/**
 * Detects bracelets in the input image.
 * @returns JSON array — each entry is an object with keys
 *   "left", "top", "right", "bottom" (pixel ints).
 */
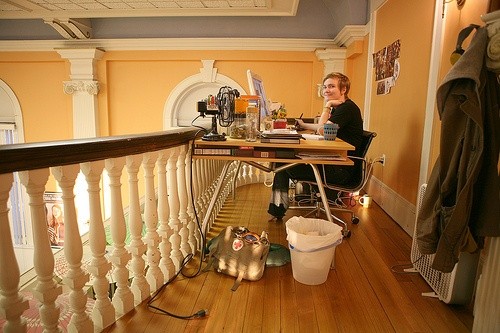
[{"left": 324, "top": 107, "right": 331, "bottom": 114}]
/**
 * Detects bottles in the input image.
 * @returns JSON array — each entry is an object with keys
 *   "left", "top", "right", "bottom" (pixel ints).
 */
[
  {"left": 246, "top": 103, "right": 259, "bottom": 142},
  {"left": 229, "top": 118, "right": 246, "bottom": 139}
]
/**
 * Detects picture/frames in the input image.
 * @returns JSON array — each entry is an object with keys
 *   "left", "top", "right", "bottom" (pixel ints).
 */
[{"left": 43, "top": 193, "right": 64, "bottom": 249}]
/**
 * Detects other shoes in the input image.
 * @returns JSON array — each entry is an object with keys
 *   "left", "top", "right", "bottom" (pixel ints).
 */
[{"left": 267, "top": 202, "right": 286, "bottom": 220}]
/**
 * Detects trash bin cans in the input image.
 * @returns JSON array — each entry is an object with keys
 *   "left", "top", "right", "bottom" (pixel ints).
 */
[{"left": 285, "top": 215, "right": 344, "bottom": 286}]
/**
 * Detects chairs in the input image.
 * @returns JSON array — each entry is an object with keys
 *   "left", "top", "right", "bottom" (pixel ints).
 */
[{"left": 268, "top": 130, "right": 377, "bottom": 239}]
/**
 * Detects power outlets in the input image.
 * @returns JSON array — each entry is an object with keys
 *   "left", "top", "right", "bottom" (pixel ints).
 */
[{"left": 380, "top": 154, "right": 386, "bottom": 166}]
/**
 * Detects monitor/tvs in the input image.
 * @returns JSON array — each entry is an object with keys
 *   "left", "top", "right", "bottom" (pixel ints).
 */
[{"left": 247, "top": 70, "right": 270, "bottom": 122}]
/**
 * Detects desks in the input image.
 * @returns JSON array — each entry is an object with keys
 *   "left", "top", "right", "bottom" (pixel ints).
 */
[{"left": 190, "top": 129, "right": 355, "bottom": 262}]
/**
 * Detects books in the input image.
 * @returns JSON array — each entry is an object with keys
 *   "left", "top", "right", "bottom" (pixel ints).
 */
[{"left": 261, "top": 133, "right": 302, "bottom": 143}]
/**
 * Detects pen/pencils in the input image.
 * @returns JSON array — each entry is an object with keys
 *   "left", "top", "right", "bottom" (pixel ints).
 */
[{"left": 296, "top": 113, "right": 303, "bottom": 125}]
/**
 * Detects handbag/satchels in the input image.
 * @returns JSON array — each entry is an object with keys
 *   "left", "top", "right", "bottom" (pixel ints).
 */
[{"left": 205, "top": 225, "right": 271, "bottom": 281}]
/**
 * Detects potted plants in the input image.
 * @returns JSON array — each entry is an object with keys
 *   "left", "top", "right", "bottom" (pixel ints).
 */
[{"left": 272, "top": 106, "right": 287, "bottom": 129}]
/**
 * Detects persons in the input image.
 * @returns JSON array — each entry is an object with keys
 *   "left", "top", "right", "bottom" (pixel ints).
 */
[
  {"left": 50, "top": 205, "right": 65, "bottom": 241},
  {"left": 44, "top": 207, "right": 60, "bottom": 244},
  {"left": 267, "top": 72, "right": 364, "bottom": 222}
]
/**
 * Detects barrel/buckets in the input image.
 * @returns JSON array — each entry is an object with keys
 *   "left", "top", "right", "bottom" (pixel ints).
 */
[{"left": 285, "top": 218, "right": 342, "bottom": 285}]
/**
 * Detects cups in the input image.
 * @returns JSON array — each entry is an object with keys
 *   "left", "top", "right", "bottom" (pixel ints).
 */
[
  {"left": 274, "top": 120, "right": 288, "bottom": 130},
  {"left": 318, "top": 124, "right": 337, "bottom": 141},
  {"left": 359, "top": 194, "right": 372, "bottom": 209}
]
